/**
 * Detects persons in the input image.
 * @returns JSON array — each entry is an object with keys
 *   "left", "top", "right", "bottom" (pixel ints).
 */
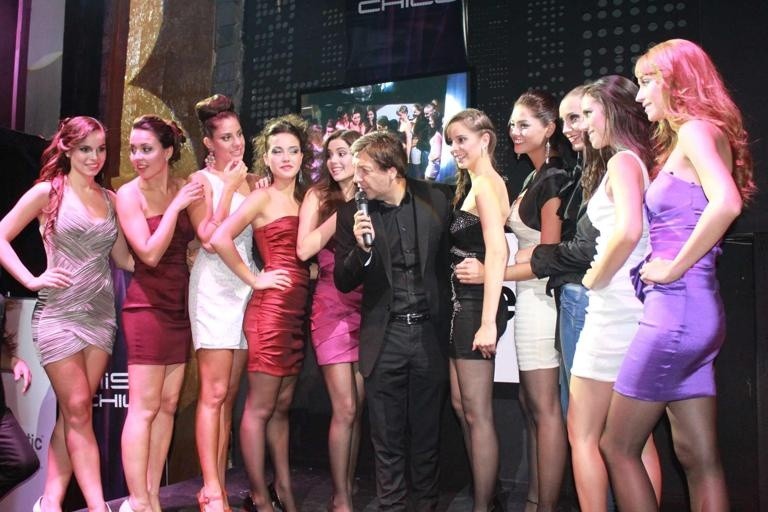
[{"left": 1, "top": 38, "right": 757, "bottom": 512}]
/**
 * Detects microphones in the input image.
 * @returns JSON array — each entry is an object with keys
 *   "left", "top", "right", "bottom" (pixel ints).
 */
[{"left": 353, "top": 190, "right": 375, "bottom": 249}]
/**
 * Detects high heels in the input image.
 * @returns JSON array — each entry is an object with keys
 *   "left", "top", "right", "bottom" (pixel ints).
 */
[
  {"left": 196, "top": 485, "right": 233, "bottom": 512},
  {"left": 267, "top": 483, "right": 286, "bottom": 512},
  {"left": 244, "top": 493, "right": 260, "bottom": 512}
]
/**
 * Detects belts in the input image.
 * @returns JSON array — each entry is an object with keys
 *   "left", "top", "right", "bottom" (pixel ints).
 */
[{"left": 391, "top": 311, "right": 432, "bottom": 325}]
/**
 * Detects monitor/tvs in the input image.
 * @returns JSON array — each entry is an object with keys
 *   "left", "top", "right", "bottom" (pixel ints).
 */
[{"left": 294, "top": 66, "right": 474, "bottom": 194}]
